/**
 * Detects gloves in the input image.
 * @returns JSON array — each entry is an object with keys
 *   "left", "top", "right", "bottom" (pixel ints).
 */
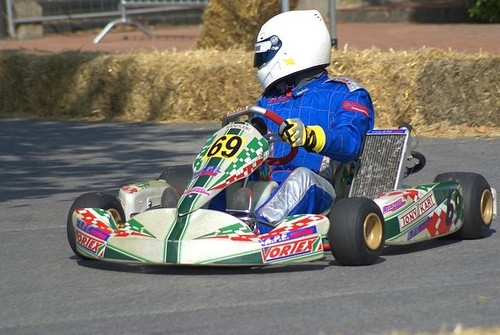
[
  {"left": 279, "top": 118, "right": 326, "bottom": 154},
  {"left": 236, "top": 112, "right": 249, "bottom": 122}
]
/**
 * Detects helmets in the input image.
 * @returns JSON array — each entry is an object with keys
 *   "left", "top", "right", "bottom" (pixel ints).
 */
[{"left": 253, "top": 10, "right": 332, "bottom": 92}]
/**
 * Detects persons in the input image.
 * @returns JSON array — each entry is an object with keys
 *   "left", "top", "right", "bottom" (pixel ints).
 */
[{"left": 200, "top": 9, "right": 375, "bottom": 235}]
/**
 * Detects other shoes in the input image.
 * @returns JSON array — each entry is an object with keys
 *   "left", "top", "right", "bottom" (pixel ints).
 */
[
  {"left": 161, "top": 187, "right": 178, "bottom": 208},
  {"left": 229, "top": 187, "right": 255, "bottom": 232}
]
